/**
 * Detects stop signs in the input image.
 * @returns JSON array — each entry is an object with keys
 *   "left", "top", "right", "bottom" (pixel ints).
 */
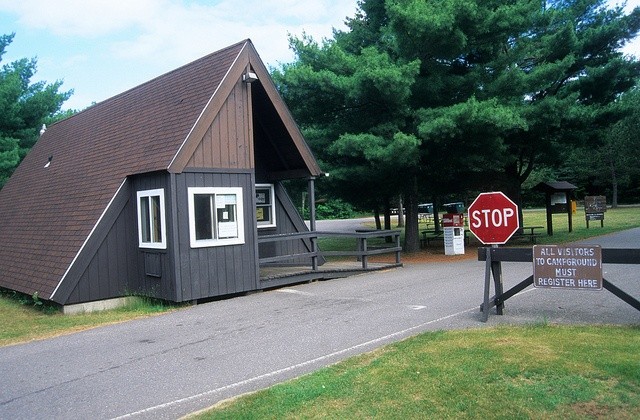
[{"left": 468, "top": 191, "right": 519, "bottom": 244}]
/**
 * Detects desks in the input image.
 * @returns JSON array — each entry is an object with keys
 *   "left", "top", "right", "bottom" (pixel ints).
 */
[
  {"left": 420, "top": 230, "right": 470, "bottom": 237},
  {"left": 518, "top": 226, "right": 544, "bottom": 234}
]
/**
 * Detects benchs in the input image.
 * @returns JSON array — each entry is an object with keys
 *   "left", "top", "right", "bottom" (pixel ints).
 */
[
  {"left": 420, "top": 238, "right": 465, "bottom": 249},
  {"left": 426, "top": 222, "right": 444, "bottom": 230},
  {"left": 509, "top": 234, "right": 543, "bottom": 245}
]
